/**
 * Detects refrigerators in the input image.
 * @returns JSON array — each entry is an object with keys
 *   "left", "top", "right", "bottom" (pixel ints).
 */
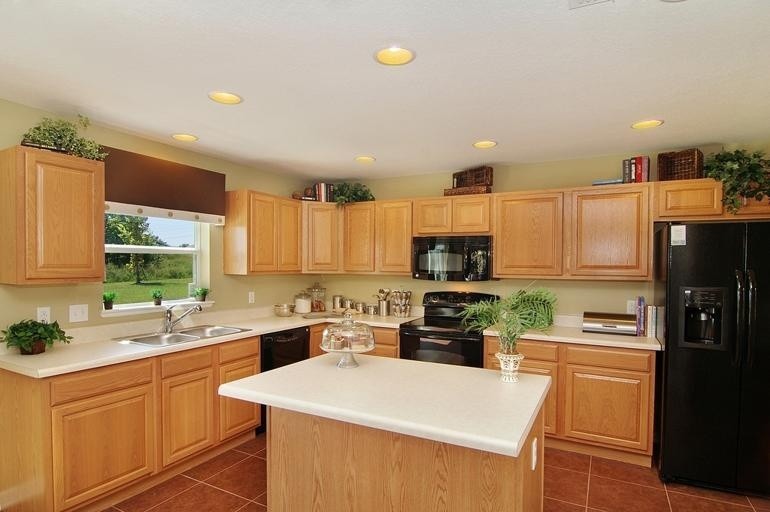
[{"left": 651, "top": 221, "right": 770, "bottom": 498}]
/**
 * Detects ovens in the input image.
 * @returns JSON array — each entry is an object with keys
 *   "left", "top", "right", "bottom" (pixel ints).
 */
[{"left": 398, "top": 332, "right": 483, "bottom": 367}]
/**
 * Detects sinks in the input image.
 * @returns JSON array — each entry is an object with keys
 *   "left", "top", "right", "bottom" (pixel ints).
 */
[
  {"left": 177, "top": 325, "right": 253, "bottom": 344},
  {"left": 111, "top": 331, "right": 200, "bottom": 354}
]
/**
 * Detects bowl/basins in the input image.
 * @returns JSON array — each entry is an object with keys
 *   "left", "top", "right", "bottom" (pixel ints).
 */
[{"left": 274, "top": 304, "right": 296, "bottom": 316}]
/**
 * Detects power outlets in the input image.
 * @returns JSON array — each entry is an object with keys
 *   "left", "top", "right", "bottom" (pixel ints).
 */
[{"left": 36, "top": 307, "right": 50, "bottom": 324}]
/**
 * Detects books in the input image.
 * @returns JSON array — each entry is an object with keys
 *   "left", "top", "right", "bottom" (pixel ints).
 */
[
  {"left": 299, "top": 182, "right": 334, "bottom": 203},
  {"left": 636, "top": 295, "right": 656, "bottom": 338},
  {"left": 622, "top": 155, "right": 650, "bottom": 183},
  {"left": 21, "top": 142, "right": 66, "bottom": 152},
  {"left": 592, "top": 179, "right": 622, "bottom": 186}
]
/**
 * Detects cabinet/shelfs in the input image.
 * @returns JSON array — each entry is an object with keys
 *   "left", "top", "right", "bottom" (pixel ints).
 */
[
  {"left": 0, "top": 358, "right": 158, "bottom": 512},
  {"left": 223, "top": 189, "right": 305, "bottom": 276},
  {"left": 483, "top": 336, "right": 656, "bottom": 457},
  {"left": 310, "top": 324, "right": 398, "bottom": 359},
  {"left": 0, "top": 145, "right": 105, "bottom": 286},
  {"left": 305, "top": 200, "right": 344, "bottom": 275},
  {"left": 158, "top": 337, "right": 261, "bottom": 468}
]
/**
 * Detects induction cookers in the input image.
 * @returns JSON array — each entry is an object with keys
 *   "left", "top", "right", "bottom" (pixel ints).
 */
[{"left": 400, "top": 316, "right": 483, "bottom": 336}]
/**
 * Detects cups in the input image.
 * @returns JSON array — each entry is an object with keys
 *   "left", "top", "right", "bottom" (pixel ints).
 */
[{"left": 377, "top": 300, "right": 390, "bottom": 315}]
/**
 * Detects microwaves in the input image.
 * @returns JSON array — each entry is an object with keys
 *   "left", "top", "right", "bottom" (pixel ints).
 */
[{"left": 411, "top": 235, "right": 500, "bottom": 282}]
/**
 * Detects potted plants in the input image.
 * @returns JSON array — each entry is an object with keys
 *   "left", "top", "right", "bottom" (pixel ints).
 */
[
  {"left": 0, "top": 320, "right": 73, "bottom": 355},
  {"left": 454, "top": 288, "right": 558, "bottom": 384},
  {"left": 103, "top": 292, "right": 116, "bottom": 310},
  {"left": 151, "top": 289, "right": 163, "bottom": 305},
  {"left": 191, "top": 288, "right": 208, "bottom": 301}
]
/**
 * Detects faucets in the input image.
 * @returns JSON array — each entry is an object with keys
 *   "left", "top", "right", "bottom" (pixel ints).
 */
[{"left": 165, "top": 304, "right": 203, "bottom": 333}]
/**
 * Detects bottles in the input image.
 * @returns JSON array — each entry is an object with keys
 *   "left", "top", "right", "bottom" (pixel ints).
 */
[
  {"left": 307, "top": 281, "right": 327, "bottom": 313},
  {"left": 392, "top": 290, "right": 411, "bottom": 317},
  {"left": 294, "top": 290, "right": 312, "bottom": 314},
  {"left": 332, "top": 294, "right": 378, "bottom": 316}
]
